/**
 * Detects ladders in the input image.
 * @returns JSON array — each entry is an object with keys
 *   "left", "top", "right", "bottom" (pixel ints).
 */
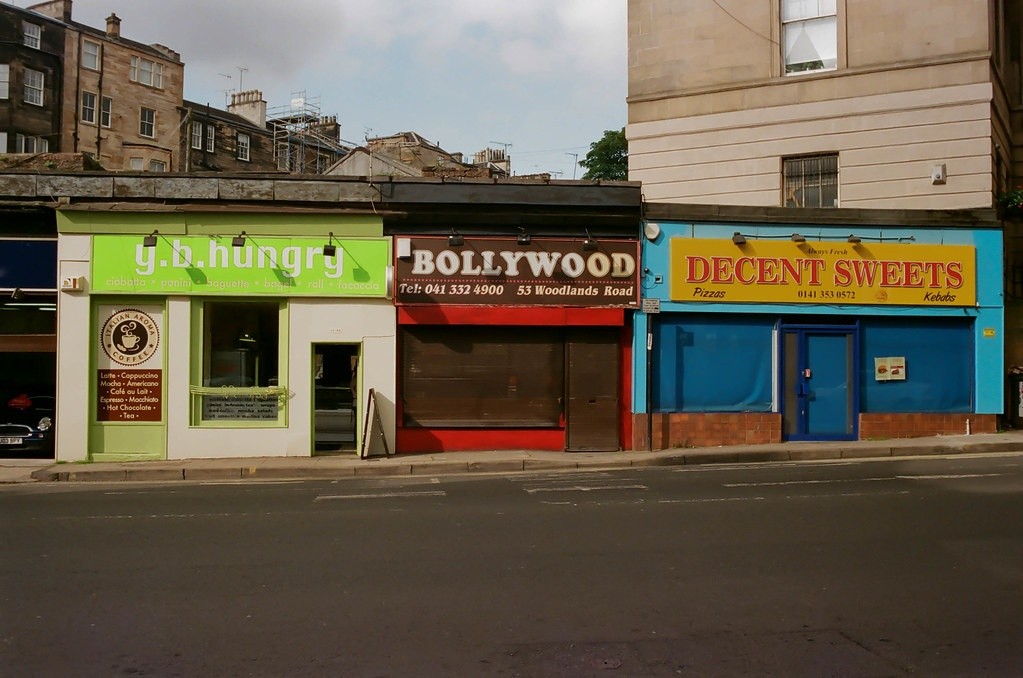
[{"left": 360, "top": 388, "right": 392, "bottom": 460}]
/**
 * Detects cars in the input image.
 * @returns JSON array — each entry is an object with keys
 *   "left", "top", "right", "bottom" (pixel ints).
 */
[{"left": 0, "top": 396, "right": 56, "bottom": 451}]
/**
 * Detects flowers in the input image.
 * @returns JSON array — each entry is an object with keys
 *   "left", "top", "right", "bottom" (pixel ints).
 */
[{"left": 998, "top": 184, "right": 1023, "bottom": 209}]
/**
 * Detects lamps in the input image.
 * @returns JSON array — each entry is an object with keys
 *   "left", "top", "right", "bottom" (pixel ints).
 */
[{"left": 143, "top": 222, "right": 862, "bottom": 256}]
